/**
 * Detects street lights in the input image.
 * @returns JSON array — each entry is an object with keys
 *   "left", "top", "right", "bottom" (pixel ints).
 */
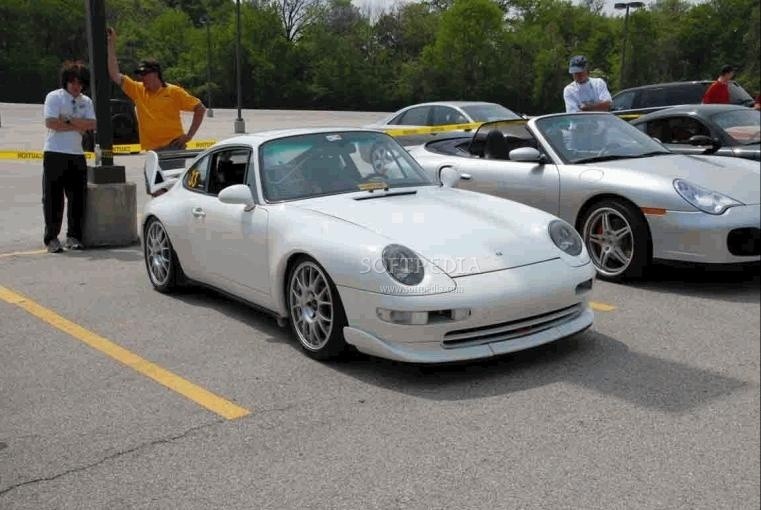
[{"left": 615, "top": 2, "right": 645, "bottom": 81}]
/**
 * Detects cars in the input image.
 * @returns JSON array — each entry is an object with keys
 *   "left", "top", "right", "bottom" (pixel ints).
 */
[{"left": 140, "top": 80, "right": 761, "bottom": 363}]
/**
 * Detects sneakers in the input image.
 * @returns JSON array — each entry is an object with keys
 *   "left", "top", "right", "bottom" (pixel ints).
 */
[
  {"left": 66, "top": 237, "right": 85, "bottom": 251},
  {"left": 47, "top": 236, "right": 65, "bottom": 253}
]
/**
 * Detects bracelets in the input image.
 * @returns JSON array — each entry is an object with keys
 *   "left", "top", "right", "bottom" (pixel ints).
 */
[{"left": 65, "top": 116, "right": 71, "bottom": 124}]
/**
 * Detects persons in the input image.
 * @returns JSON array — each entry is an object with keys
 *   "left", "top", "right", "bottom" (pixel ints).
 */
[
  {"left": 106, "top": 26, "right": 208, "bottom": 196},
  {"left": 702, "top": 64, "right": 740, "bottom": 104},
  {"left": 41, "top": 64, "right": 98, "bottom": 254},
  {"left": 563, "top": 55, "right": 614, "bottom": 150},
  {"left": 755, "top": 90, "right": 761, "bottom": 111}
]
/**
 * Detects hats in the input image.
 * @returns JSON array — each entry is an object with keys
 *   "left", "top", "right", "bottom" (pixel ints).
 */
[
  {"left": 132, "top": 58, "right": 162, "bottom": 75},
  {"left": 567, "top": 55, "right": 591, "bottom": 74}
]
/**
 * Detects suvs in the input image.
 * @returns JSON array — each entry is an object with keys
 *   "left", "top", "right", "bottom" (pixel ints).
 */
[{"left": 82, "top": 98, "right": 139, "bottom": 151}]
